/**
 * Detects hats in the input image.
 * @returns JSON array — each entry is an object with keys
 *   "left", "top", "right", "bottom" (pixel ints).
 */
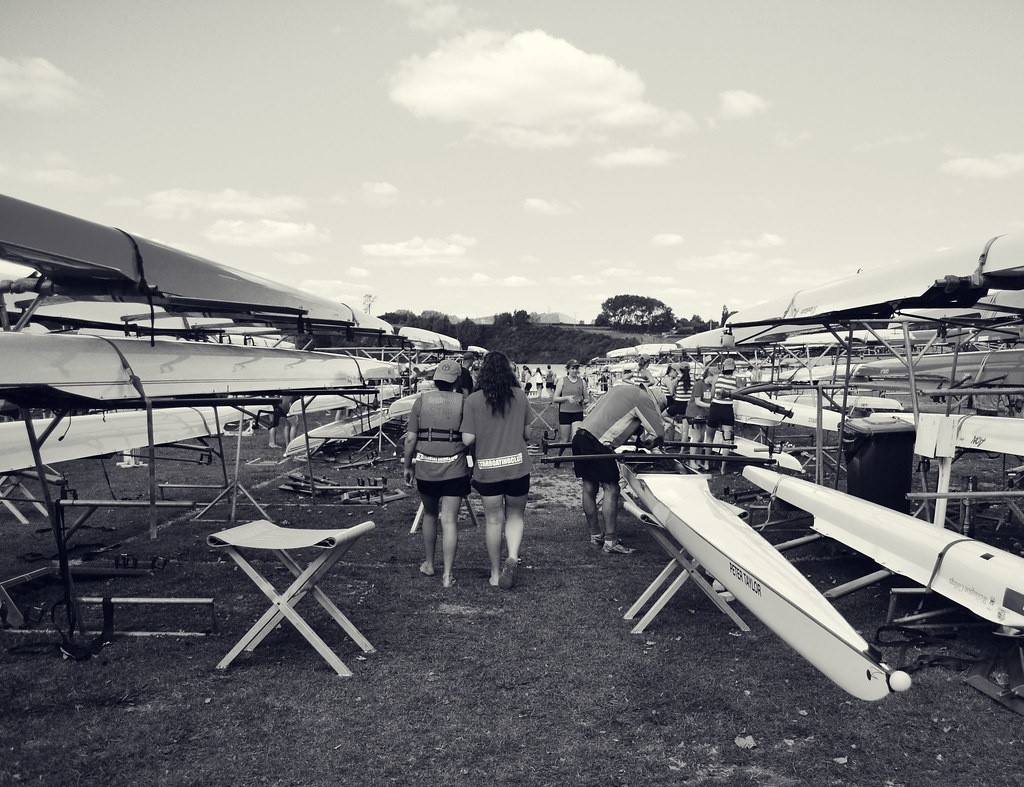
[
  {"left": 639, "top": 354, "right": 735, "bottom": 376},
  {"left": 566, "top": 359, "right": 580, "bottom": 366},
  {"left": 433, "top": 360, "right": 461, "bottom": 383},
  {"left": 641, "top": 384, "right": 667, "bottom": 418},
  {"left": 464, "top": 353, "right": 475, "bottom": 361}
]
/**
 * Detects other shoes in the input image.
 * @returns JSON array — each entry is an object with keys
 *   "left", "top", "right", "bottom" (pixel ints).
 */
[
  {"left": 420, "top": 562, "right": 435, "bottom": 576},
  {"left": 442, "top": 573, "right": 456, "bottom": 587}
]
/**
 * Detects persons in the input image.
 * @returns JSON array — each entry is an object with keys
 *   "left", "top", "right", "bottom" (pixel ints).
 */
[
  {"left": 571, "top": 381, "right": 669, "bottom": 556},
  {"left": 701, "top": 357, "right": 746, "bottom": 475},
  {"left": 402, "top": 359, "right": 474, "bottom": 588},
  {"left": 552, "top": 358, "right": 589, "bottom": 469},
  {"left": 500, "top": 350, "right": 764, "bottom": 410},
  {"left": 683, "top": 366, "right": 723, "bottom": 472},
  {"left": 264, "top": 385, "right": 305, "bottom": 454},
  {"left": 458, "top": 351, "right": 536, "bottom": 586},
  {"left": 453, "top": 350, "right": 477, "bottom": 404},
  {"left": 677, "top": 373, "right": 712, "bottom": 465}
]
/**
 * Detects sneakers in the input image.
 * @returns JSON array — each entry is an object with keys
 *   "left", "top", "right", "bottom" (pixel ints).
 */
[
  {"left": 498, "top": 555, "right": 518, "bottom": 589},
  {"left": 488, "top": 571, "right": 499, "bottom": 586},
  {"left": 602, "top": 539, "right": 637, "bottom": 556},
  {"left": 590, "top": 531, "right": 605, "bottom": 549}
]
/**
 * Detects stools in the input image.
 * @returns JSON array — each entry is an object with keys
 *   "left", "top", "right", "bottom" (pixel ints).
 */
[
  {"left": 200, "top": 518, "right": 377, "bottom": 677},
  {"left": 527, "top": 400, "right": 555, "bottom": 430}
]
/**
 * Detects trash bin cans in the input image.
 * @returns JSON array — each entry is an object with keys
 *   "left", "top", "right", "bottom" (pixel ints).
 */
[{"left": 838, "top": 412, "right": 917, "bottom": 516}]
